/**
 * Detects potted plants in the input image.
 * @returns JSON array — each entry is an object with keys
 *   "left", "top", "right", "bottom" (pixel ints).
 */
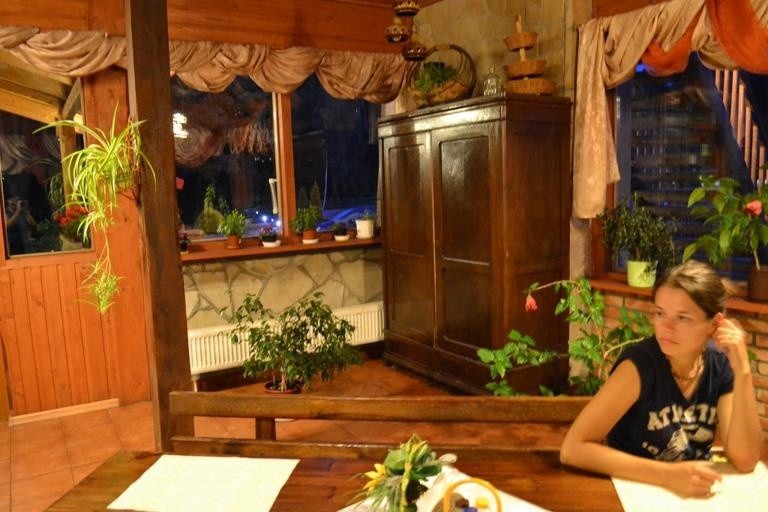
[
  {"left": 590, "top": 188, "right": 686, "bottom": 289},
  {"left": 212, "top": 288, "right": 366, "bottom": 422},
  {"left": 30, "top": 95, "right": 159, "bottom": 317},
  {"left": 214, "top": 199, "right": 377, "bottom": 252}
]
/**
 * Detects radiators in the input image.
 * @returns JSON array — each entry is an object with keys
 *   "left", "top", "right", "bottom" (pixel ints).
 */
[{"left": 186, "top": 302, "right": 385, "bottom": 392}]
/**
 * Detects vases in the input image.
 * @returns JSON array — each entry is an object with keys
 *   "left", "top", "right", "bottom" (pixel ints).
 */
[{"left": 747, "top": 264, "right": 768, "bottom": 304}]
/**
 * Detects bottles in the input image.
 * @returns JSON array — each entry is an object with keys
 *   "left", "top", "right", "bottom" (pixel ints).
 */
[{"left": 482, "top": 66, "right": 503, "bottom": 98}]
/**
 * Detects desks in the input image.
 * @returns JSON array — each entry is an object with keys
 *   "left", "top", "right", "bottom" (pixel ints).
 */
[{"left": 40, "top": 450, "right": 768, "bottom": 512}]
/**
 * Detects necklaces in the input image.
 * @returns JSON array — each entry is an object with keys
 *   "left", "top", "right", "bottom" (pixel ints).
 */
[{"left": 669, "top": 355, "right": 703, "bottom": 381}]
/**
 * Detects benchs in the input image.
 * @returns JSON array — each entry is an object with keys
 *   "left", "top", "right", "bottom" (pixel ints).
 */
[{"left": 168, "top": 390, "right": 606, "bottom": 459}]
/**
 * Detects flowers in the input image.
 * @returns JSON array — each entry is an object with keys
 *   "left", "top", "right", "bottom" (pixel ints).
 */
[
  {"left": 475, "top": 275, "right": 656, "bottom": 399},
  {"left": 683, "top": 173, "right": 768, "bottom": 269}
]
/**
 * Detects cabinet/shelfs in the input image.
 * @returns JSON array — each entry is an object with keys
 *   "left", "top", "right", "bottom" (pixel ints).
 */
[{"left": 377, "top": 91, "right": 577, "bottom": 396}]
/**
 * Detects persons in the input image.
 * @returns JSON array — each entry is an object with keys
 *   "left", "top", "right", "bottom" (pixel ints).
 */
[
  {"left": 558, "top": 258, "right": 764, "bottom": 499},
  {"left": 6, "top": 191, "right": 38, "bottom": 252}
]
